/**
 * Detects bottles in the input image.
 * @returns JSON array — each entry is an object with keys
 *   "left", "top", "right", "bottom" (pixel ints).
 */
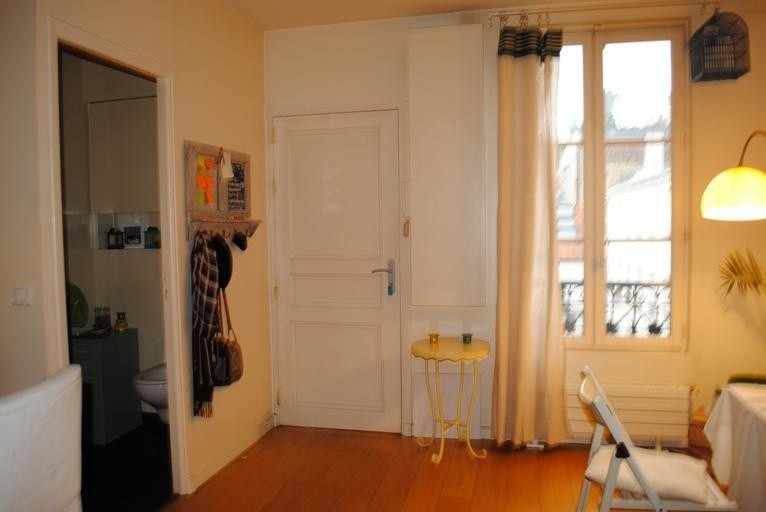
[{"left": 92, "top": 303, "right": 127, "bottom": 335}]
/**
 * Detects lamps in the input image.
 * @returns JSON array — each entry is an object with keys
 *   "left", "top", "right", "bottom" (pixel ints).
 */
[{"left": 700, "top": 123, "right": 766, "bottom": 221}]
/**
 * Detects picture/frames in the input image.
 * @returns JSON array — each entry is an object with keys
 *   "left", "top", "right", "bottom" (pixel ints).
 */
[{"left": 183, "top": 140, "right": 254, "bottom": 221}]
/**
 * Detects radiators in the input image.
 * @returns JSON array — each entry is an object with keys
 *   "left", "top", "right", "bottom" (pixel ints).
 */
[{"left": 563, "top": 378, "right": 691, "bottom": 448}]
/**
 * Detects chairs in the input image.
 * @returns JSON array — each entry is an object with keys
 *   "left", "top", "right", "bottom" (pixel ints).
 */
[{"left": 574, "top": 362, "right": 739, "bottom": 512}]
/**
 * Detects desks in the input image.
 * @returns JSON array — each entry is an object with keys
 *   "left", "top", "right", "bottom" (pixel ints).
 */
[
  {"left": 409, "top": 334, "right": 496, "bottom": 466},
  {"left": 700, "top": 378, "right": 765, "bottom": 512}
]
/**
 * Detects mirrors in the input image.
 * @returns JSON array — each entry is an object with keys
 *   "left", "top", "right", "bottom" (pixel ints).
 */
[{"left": 83, "top": 93, "right": 160, "bottom": 246}]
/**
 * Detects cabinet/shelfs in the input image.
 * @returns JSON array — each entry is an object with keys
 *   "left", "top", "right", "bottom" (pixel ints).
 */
[{"left": 66, "top": 324, "right": 143, "bottom": 451}]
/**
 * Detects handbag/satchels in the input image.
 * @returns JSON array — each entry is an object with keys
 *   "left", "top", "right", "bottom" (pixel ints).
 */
[{"left": 211, "top": 336, "right": 244, "bottom": 386}]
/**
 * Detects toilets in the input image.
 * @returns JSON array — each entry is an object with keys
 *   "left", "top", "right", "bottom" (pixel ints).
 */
[{"left": 131, "top": 363, "right": 170, "bottom": 449}]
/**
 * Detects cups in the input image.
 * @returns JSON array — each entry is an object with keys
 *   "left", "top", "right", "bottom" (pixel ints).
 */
[
  {"left": 428, "top": 333, "right": 439, "bottom": 345},
  {"left": 462, "top": 334, "right": 474, "bottom": 345}
]
